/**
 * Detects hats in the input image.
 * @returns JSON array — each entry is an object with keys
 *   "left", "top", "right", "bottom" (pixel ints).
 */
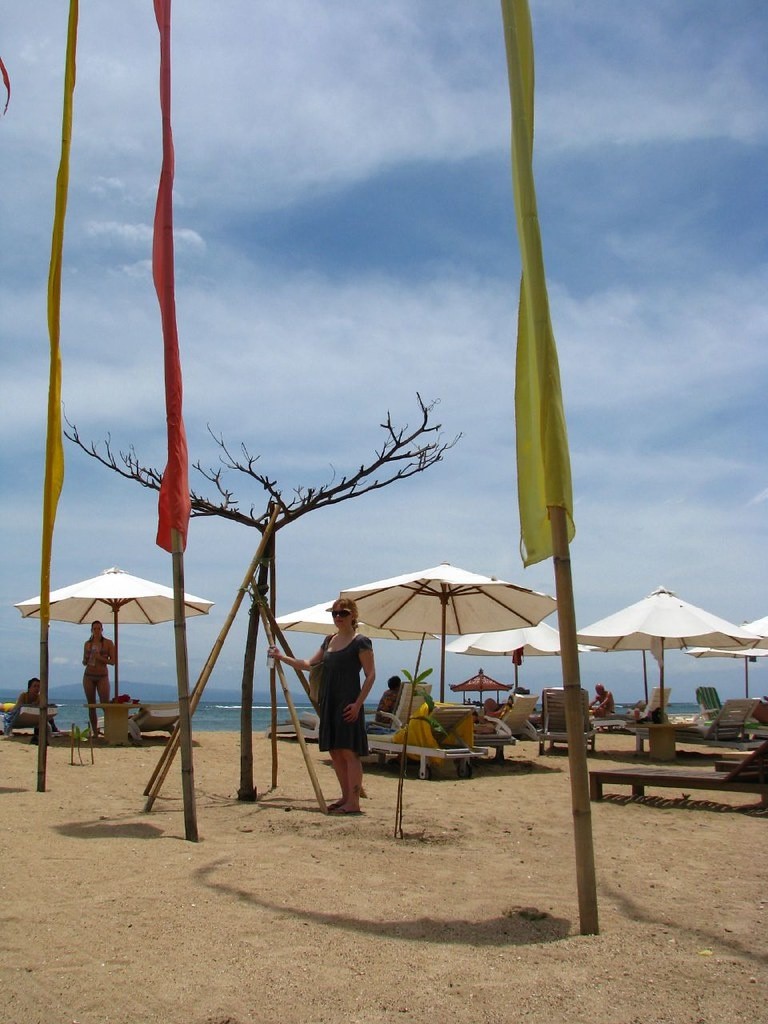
[
  {"left": 388, "top": 676, "right": 401, "bottom": 687},
  {"left": 514, "top": 687, "right": 530, "bottom": 695}
]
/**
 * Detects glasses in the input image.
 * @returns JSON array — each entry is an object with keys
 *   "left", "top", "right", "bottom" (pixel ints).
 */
[{"left": 332, "top": 610, "right": 353, "bottom": 617}]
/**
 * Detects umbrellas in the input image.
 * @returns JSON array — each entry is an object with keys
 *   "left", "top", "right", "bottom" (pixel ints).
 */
[
  {"left": 586, "top": 644, "right": 650, "bottom": 706},
  {"left": 444, "top": 623, "right": 592, "bottom": 658},
  {"left": 267, "top": 597, "right": 440, "bottom": 641},
  {"left": 326, "top": 561, "right": 558, "bottom": 703},
  {"left": 739, "top": 614, "right": 768, "bottom": 649},
  {"left": 14, "top": 567, "right": 215, "bottom": 698},
  {"left": 684, "top": 645, "right": 768, "bottom": 699},
  {"left": 575, "top": 585, "right": 765, "bottom": 723}
]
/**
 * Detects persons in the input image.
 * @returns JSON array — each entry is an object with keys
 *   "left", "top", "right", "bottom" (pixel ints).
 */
[
  {"left": 588, "top": 684, "right": 616, "bottom": 732},
  {"left": 267, "top": 597, "right": 375, "bottom": 816},
  {"left": 374, "top": 676, "right": 402, "bottom": 724},
  {"left": 632, "top": 707, "right": 661, "bottom": 723},
  {"left": 80, "top": 620, "right": 116, "bottom": 738},
  {"left": 467, "top": 697, "right": 511, "bottom": 723},
  {"left": 0, "top": 678, "right": 41, "bottom": 713}
]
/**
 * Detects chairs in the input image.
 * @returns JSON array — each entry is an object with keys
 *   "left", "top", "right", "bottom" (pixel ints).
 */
[
  {"left": 474, "top": 729, "right": 516, "bottom": 764},
  {"left": 367, "top": 701, "right": 496, "bottom": 780},
  {"left": 539, "top": 687, "right": 597, "bottom": 755},
  {"left": 366, "top": 682, "right": 432, "bottom": 730},
  {"left": 97, "top": 705, "right": 179, "bottom": 740},
  {"left": 266, "top": 712, "right": 319, "bottom": 739},
  {"left": 589, "top": 739, "right": 768, "bottom": 802},
  {"left": 635, "top": 698, "right": 768, "bottom": 752},
  {"left": 495, "top": 694, "right": 539, "bottom": 735},
  {"left": 0, "top": 703, "right": 58, "bottom": 746},
  {"left": 592, "top": 687, "right": 671, "bottom": 732},
  {"left": 696, "top": 685, "right": 768, "bottom": 736}
]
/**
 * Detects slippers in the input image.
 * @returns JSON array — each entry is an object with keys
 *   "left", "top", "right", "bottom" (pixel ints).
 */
[
  {"left": 332, "top": 809, "right": 361, "bottom": 815},
  {"left": 327, "top": 802, "right": 340, "bottom": 811}
]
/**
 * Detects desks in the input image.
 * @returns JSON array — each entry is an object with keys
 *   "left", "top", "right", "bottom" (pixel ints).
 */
[{"left": 84, "top": 703, "right": 141, "bottom": 745}]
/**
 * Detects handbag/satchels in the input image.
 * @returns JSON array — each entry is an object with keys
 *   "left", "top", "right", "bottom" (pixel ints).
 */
[{"left": 308, "top": 633, "right": 337, "bottom": 704}]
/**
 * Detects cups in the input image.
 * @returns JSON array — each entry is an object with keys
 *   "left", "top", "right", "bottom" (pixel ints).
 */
[{"left": 634, "top": 710, "right": 640, "bottom": 721}]
